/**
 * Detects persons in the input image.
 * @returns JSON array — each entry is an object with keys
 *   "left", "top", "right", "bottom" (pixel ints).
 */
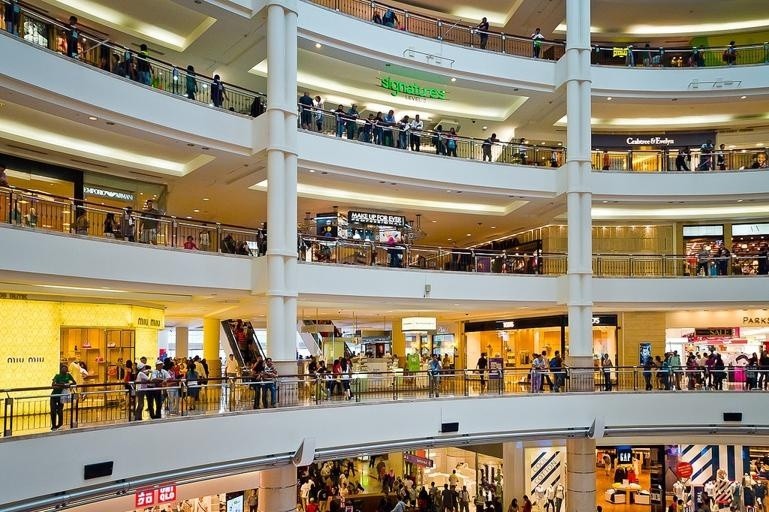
[
  {"left": 676, "top": 141, "right": 727, "bottom": 171},
  {"left": 308, "top": 350, "right": 566, "bottom": 402},
  {"left": 481, "top": 133, "right": 499, "bottom": 161},
  {"left": 50, "top": 318, "right": 279, "bottom": 431},
  {"left": 602, "top": 451, "right": 768, "bottom": 511},
  {"left": 251, "top": 91, "right": 458, "bottom": 157},
  {"left": 477, "top": 17, "right": 489, "bottom": 50},
  {"left": 372, "top": 9, "right": 400, "bottom": 28},
  {"left": 299, "top": 227, "right": 542, "bottom": 275},
  {"left": 551, "top": 148, "right": 559, "bottom": 167},
  {"left": 4, "top": 1, "right": 230, "bottom": 106},
  {"left": 601, "top": 149, "right": 610, "bottom": 170},
  {"left": 751, "top": 153, "right": 767, "bottom": 169},
  {"left": 518, "top": 138, "right": 528, "bottom": 165},
  {"left": 695, "top": 242, "right": 769, "bottom": 277},
  {"left": 592, "top": 40, "right": 738, "bottom": 67},
  {"left": 531, "top": 28, "right": 544, "bottom": 58},
  {"left": 249, "top": 456, "right": 564, "bottom": 511},
  {"left": 602, "top": 349, "right": 769, "bottom": 393},
  {"left": 12, "top": 196, "right": 267, "bottom": 257}
]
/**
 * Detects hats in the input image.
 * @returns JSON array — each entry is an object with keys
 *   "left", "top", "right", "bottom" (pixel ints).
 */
[
  {"left": 156, "top": 360, "right": 163, "bottom": 364},
  {"left": 137, "top": 363, "right": 144, "bottom": 370}
]
